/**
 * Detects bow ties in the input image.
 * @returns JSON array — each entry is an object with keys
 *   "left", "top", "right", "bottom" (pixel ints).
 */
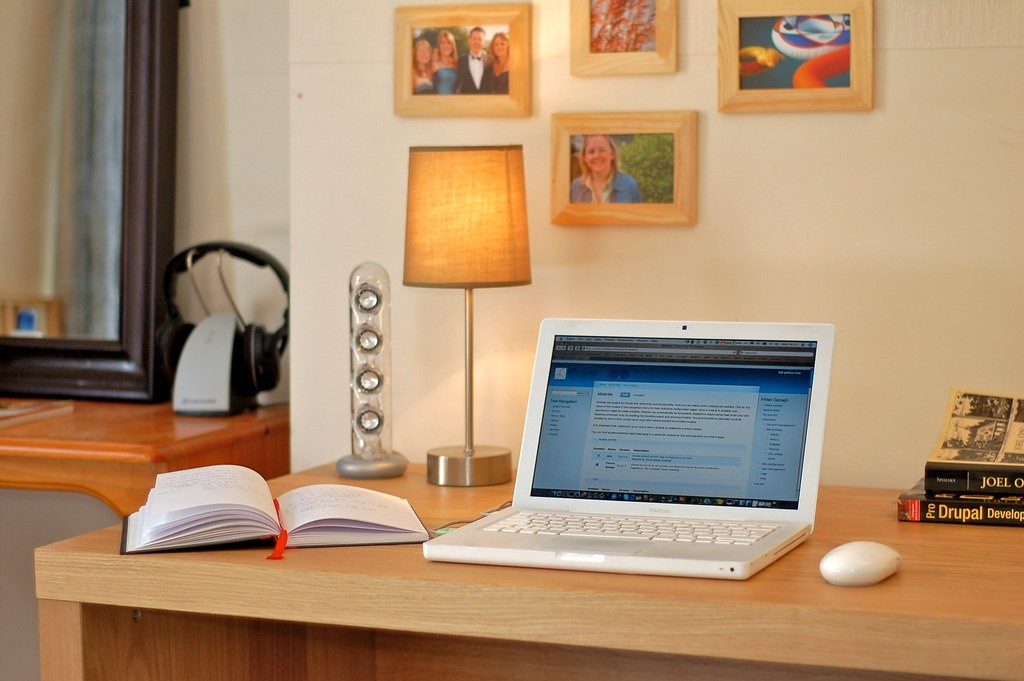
[{"left": 470, "top": 52, "right": 482, "bottom": 61}]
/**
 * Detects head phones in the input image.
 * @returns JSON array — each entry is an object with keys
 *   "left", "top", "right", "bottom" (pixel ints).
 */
[{"left": 155, "top": 241, "right": 290, "bottom": 399}]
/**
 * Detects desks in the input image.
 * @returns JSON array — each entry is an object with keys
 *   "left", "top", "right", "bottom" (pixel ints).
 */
[
  {"left": 33, "top": 460, "right": 1024, "bottom": 681},
  {"left": 0, "top": 396, "right": 291, "bottom": 520}
]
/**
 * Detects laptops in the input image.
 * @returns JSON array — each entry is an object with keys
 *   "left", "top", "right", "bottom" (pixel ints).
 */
[{"left": 424, "top": 319, "right": 835, "bottom": 581}]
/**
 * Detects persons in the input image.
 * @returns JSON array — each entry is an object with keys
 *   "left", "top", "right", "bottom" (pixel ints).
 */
[
  {"left": 413, "top": 40, "right": 433, "bottom": 95},
  {"left": 488, "top": 32, "right": 509, "bottom": 94},
  {"left": 431, "top": 30, "right": 457, "bottom": 95},
  {"left": 458, "top": 26, "right": 492, "bottom": 95},
  {"left": 570, "top": 135, "right": 643, "bottom": 204}
]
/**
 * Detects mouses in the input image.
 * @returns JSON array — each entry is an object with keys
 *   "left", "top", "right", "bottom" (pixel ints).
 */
[{"left": 819, "top": 542, "right": 901, "bottom": 587}]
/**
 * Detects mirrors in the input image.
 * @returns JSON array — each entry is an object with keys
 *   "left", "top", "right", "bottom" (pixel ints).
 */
[{"left": 1, "top": 0, "right": 178, "bottom": 400}]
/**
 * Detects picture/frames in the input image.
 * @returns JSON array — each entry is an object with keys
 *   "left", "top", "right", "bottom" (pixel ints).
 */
[
  {"left": 718, "top": 0, "right": 877, "bottom": 112},
  {"left": 550, "top": 110, "right": 699, "bottom": 227},
  {"left": 570, "top": 1, "right": 680, "bottom": 77},
  {"left": 394, "top": 1, "right": 534, "bottom": 118}
]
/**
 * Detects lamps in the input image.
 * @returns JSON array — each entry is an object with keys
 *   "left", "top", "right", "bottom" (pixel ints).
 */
[{"left": 405, "top": 145, "right": 531, "bottom": 487}]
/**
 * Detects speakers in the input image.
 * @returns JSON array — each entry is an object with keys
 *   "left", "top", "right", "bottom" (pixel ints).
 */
[{"left": 337, "top": 263, "right": 407, "bottom": 479}]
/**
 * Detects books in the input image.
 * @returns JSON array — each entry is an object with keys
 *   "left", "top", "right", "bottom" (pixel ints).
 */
[
  {"left": 897, "top": 387, "right": 1024, "bottom": 525},
  {"left": 119, "top": 463, "right": 433, "bottom": 557}
]
